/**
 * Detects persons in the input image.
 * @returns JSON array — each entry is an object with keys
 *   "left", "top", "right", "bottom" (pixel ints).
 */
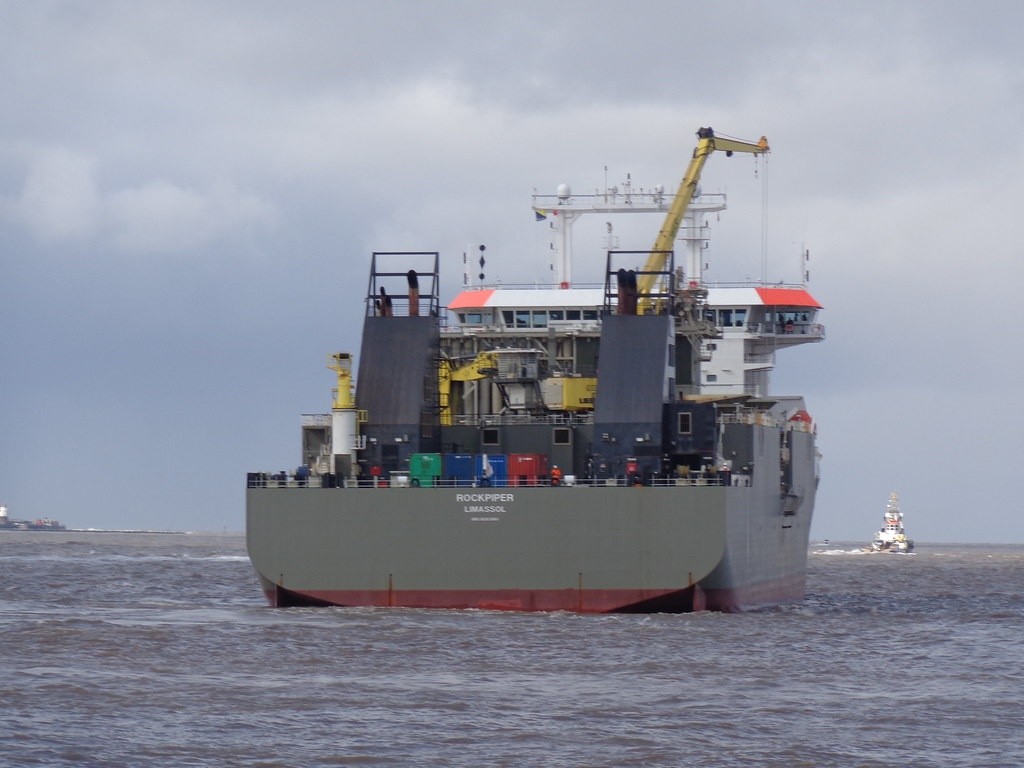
[{"left": 551, "top": 465, "right": 561, "bottom": 485}]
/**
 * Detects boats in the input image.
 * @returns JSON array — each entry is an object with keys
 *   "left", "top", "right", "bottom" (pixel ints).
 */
[
  {"left": 246, "top": 126, "right": 828, "bottom": 614},
  {"left": 871, "top": 491, "right": 912, "bottom": 554}
]
